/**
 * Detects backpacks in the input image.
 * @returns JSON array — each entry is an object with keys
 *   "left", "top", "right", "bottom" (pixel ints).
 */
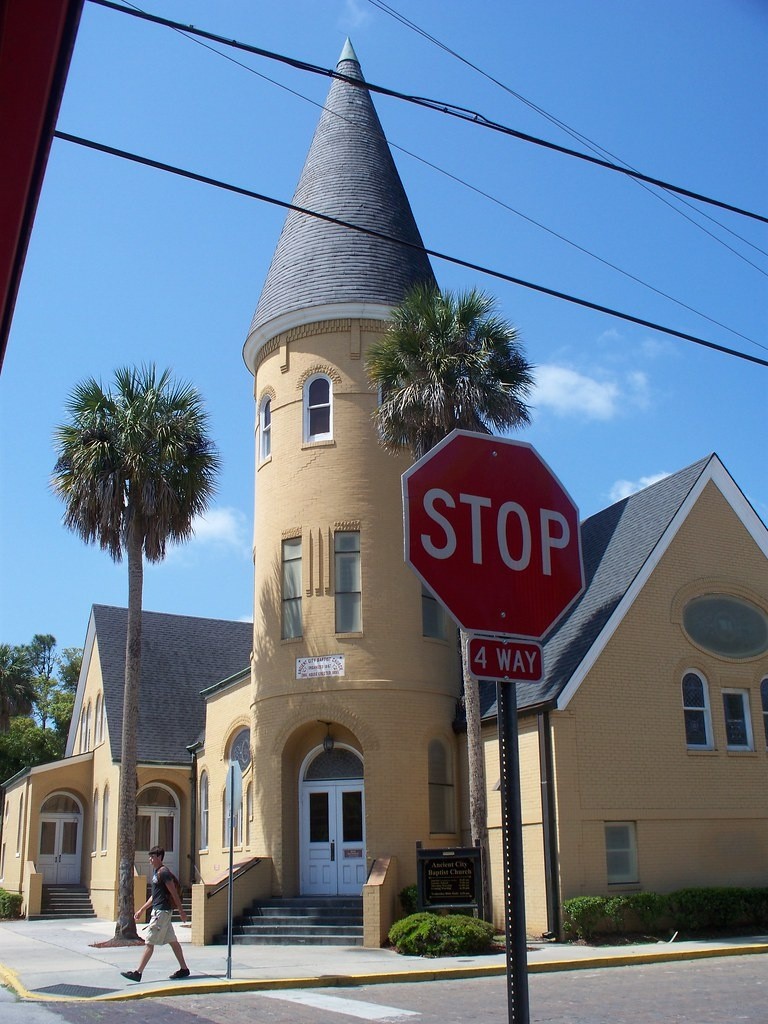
[{"left": 158, "top": 867, "right": 183, "bottom": 909}]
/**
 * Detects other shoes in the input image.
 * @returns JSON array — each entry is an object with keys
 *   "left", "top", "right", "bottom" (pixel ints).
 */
[
  {"left": 169, "top": 970, "right": 190, "bottom": 978},
  {"left": 120, "top": 970, "right": 142, "bottom": 982}
]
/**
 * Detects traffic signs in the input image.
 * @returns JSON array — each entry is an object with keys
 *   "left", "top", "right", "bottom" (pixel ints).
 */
[{"left": 401, "top": 430, "right": 587, "bottom": 643}]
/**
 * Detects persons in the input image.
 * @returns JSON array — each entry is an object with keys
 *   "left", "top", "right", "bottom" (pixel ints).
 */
[{"left": 120, "top": 846, "right": 190, "bottom": 982}]
